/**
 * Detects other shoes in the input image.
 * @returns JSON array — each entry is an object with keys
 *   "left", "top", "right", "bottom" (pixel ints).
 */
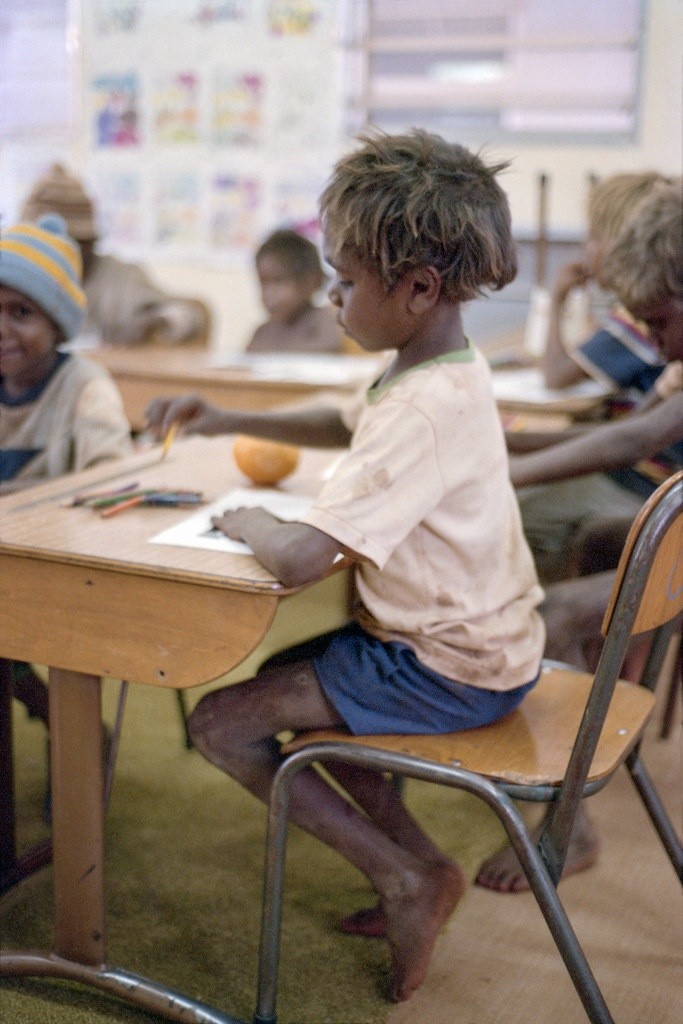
[{"left": 41, "top": 721, "right": 112, "bottom": 821}]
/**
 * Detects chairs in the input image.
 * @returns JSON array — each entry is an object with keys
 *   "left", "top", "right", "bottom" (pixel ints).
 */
[{"left": 255, "top": 470, "right": 683, "bottom": 1024}]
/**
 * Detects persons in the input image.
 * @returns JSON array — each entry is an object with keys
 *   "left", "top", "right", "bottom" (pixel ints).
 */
[
  {"left": 18, "top": 161, "right": 216, "bottom": 346},
  {"left": 240, "top": 230, "right": 364, "bottom": 359},
  {"left": 512, "top": 169, "right": 683, "bottom": 588},
  {"left": 0, "top": 212, "right": 139, "bottom": 826},
  {"left": 139, "top": 126, "right": 549, "bottom": 1004},
  {"left": 473, "top": 177, "right": 683, "bottom": 893}
]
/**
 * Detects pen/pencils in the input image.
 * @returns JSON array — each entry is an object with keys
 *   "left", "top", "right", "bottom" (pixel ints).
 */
[
  {"left": 160, "top": 421, "right": 180, "bottom": 460},
  {"left": 93, "top": 488, "right": 159, "bottom": 507},
  {"left": 60, "top": 482, "right": 138, "bottom": 508},
  {"left": 101, "top": 495, "right": 146, "bottom": 518}
]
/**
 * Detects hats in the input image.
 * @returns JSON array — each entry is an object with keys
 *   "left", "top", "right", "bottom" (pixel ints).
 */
[
  {"left": 19, "top": 166, "right": 98, "bottom": 240},
  {"left": 0, "top": 227, "right": 84, "bottom": 340}
]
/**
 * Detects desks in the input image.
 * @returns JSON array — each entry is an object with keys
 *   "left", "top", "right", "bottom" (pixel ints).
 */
[
  {"left": 481, "top": 363, "right": 640, "bottom": 438},
  {"left": 92, "top": 341, "right": 378, "bottom": 444},
  {"left": 0, "top": 438, "right": 357, "bottom": 1024}
]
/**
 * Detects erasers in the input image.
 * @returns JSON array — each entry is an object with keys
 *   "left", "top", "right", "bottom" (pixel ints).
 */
[{"left": 147, "top": 494, "right": 203, "bottom": 508}]
